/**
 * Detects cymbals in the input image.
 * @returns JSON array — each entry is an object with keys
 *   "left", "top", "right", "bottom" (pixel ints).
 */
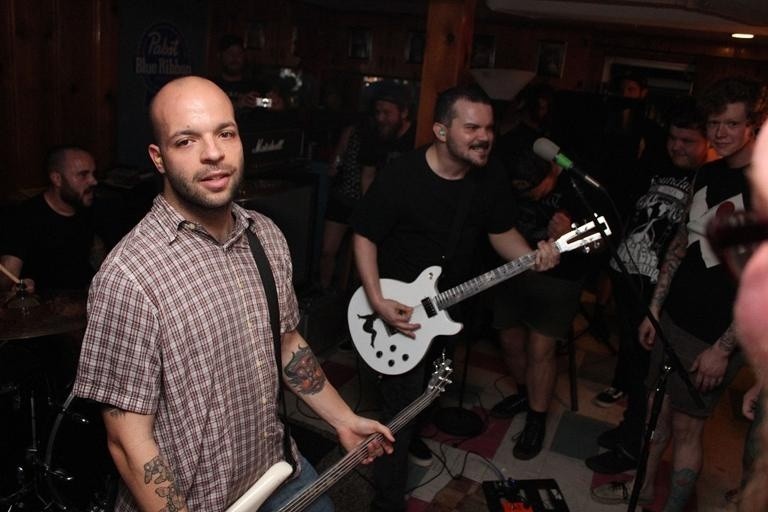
[{"left": 1, "top": 292, "right": 86, "bottom": 341}]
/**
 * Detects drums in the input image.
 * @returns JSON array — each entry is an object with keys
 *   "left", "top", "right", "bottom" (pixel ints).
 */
[{"left": 15, "top": 363, "right": 117, "bottom": 509}]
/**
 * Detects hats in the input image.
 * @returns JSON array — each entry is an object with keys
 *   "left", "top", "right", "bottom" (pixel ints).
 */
[
  {"left": 371, "top": 81, "right": 413, "bottom": 107},
  {"left": 219, "top": 33, "right": 245, "bottom": 51}
]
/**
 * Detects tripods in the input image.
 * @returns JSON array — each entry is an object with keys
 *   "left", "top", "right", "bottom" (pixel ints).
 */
[{"left": 0, "top": 397, "right": 67, "bottom": 512}]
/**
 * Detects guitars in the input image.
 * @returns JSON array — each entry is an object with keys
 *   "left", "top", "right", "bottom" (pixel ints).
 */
[
  {"left": 346, "top": 211, "right": 613, "bottom": 376},
  {"left": 224, "top": 351, "right": 453, "bottom": 512}
]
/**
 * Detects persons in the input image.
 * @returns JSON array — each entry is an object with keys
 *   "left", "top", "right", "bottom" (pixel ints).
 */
[
  {"left": 213, "top": 33, "right": 285, "bottom": 111},
  {"left": 73, "top": 76, "right": 396, "bottom": 512},
  {"left": 0, "top": 141, "right": 98, "bottom": 292},
  {"left": 306, "top": 73, "right": 767, "bottom": 512}
]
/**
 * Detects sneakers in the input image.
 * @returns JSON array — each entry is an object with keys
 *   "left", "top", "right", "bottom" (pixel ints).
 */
[
  {"left": 590, "top": 381, "right": 628, "bottom": 408},
  {"left": 488, "top": 388, "right": 530, "bottom": 419},
  {"left": 407, "top": 436, "right": 434, "bottom": 468},
  {"left": 585, "top": 448, "right": 639, "bottom": 474},
  {"left": 596, "top": 426, "right": 622, "bottom": 450},
  {"left": 590, "top": 478, "right": 658, "bottom": 506}
]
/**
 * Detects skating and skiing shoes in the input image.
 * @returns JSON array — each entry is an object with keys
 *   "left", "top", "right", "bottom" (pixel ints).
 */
[{"left": 510, "top": 411, "right": 549, "bottom": 462}]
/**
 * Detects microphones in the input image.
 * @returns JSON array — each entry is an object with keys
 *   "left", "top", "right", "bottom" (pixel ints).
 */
[{"left": 531, "top": 134, "right": 601, "bottom": 190}]
